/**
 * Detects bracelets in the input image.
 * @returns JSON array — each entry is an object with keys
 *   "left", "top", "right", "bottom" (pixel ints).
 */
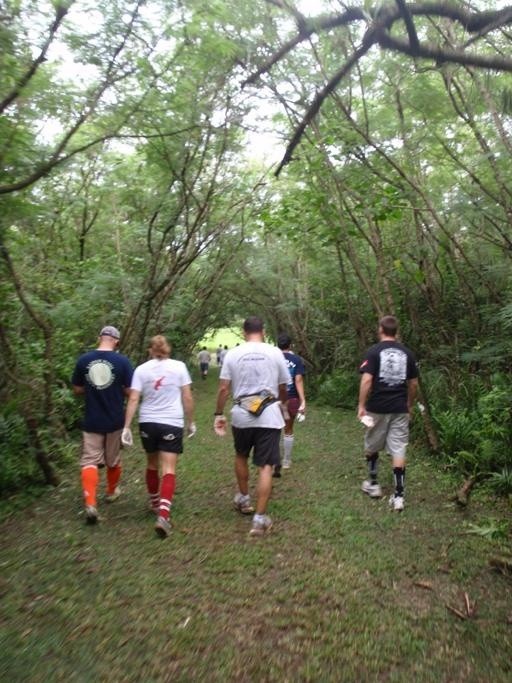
[{"left": 215, "top": 411, "right": 224, "bottom": 415}]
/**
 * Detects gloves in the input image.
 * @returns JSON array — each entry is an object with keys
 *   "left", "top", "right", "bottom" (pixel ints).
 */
[
  {"left": 213, "top": 416, "right": 227, "bottom": 435},
  {"left": 121, "top": 428, "right": 133, "bottom": 446},
  {"left": 186, "top": 425, "right": 196, "bottom": 440}
]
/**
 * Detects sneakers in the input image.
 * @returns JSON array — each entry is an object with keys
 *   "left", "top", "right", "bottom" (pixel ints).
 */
[
  {"left": 389, "top": 493, "right": 405, "bottom": 509},
  {"left": 148, "top": 504, "right": 160, "bottom": 515},
  {"left": 154, "top": 515, "right": 173, "bottom": 537},
  {"left": 233, "top": 492, "right": 255, "bottom": 513},
  {"left": 84, "top": 504, "right": 98, "bottom": 524},
  {"left": 249, "top": 516, "right": 272, "bottom": 536},
  {"left": 361, "top": 480, "right": 382, "bottom": 497},
  {"left": 104, "top": 487, "right": 121, "bottom": 504},
  {"left": 282, "top": 459, "right": 293, "bottom": 469}
]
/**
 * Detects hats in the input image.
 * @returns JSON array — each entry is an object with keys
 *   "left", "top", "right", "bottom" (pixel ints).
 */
[{"left": 99, "top": 326, "right": 121, "bottom": 347}]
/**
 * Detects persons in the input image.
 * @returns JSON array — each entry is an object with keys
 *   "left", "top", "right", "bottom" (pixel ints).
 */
[
  {"left": 272, "top": 335, "right": 306, "bottom": 477},
  {"left": 120, "top": 333, "right": 196, "bottom": 539},
  {"left": 71, "top": 326, "right": 133, "bottom": 524},
  {"left": 195, "top": 343, "right": 241, "bottom": 378},
  {"left": 213, "top": 316, "right": 293, "bottom": 534},
  {"left": 356, "top": 316, "right": 419, "bottom": 509}
]
